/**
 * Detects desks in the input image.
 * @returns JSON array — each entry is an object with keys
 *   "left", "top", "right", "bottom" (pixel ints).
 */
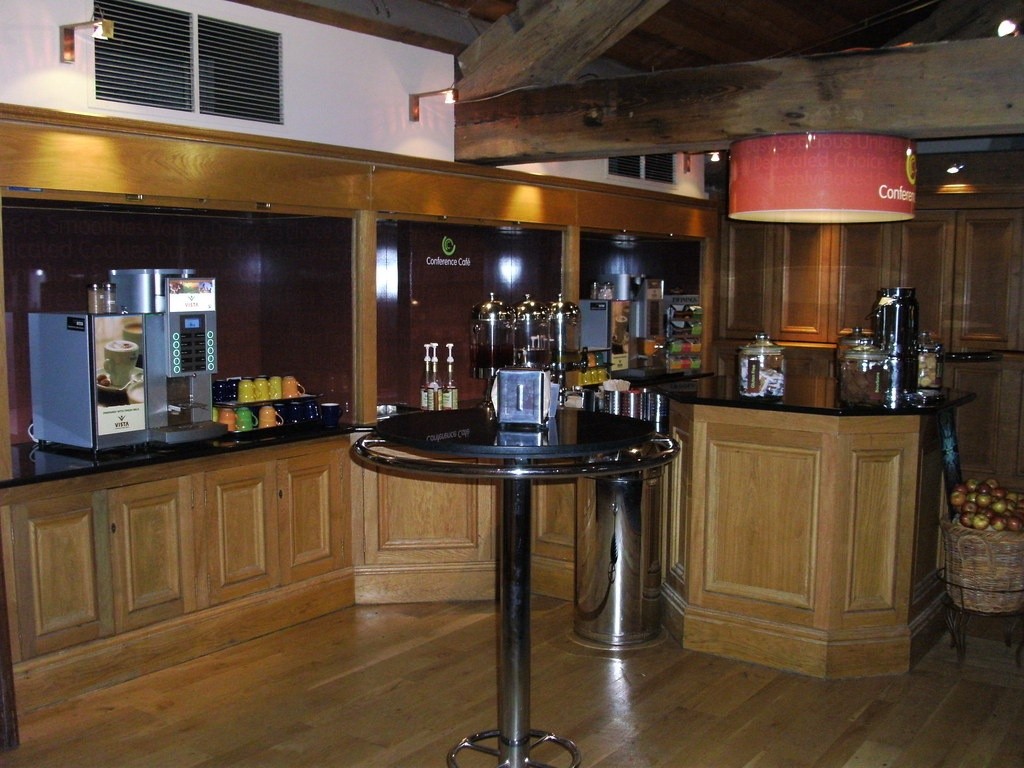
[{"left": 348, "top": 405, "right": 680, "bottom": 768}]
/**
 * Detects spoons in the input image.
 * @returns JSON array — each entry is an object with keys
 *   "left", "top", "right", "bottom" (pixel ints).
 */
[{"left": 119, "top": 372, "right": 144, "bottom": 391}]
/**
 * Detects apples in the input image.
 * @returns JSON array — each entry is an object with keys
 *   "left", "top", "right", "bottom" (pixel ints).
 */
[{"left": 950, "top": 478, "right": 1024, "bottom": 531}]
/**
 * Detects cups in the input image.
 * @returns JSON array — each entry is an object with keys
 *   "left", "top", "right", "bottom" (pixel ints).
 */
[
  {"left": 121, "top": 317, "right": 144, "bottom": 354},
  {"left": 638, "top": 335, "right": 668, "bottom": 356},
  {"left": 615, "top": 317, "right": 628, "bottom": 342},
  {"left": 211, "top": 375, "right": 320, "bottom": 432},
  {"left": 103, "top": 341, "right": 139, "bottom": 386},
  {"left": 321, "top": 403, "right": 343, "bottom": 428},
  {"left": 128, "top": 383, "right": 144, "bottom": 404},
  {"left": 566, "top": 352, "right": 612, "bottom": 388}
]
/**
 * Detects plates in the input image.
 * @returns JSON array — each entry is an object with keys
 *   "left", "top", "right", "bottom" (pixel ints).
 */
[{"left": 96, "top": 367, "right": 143, "bottom": 392}]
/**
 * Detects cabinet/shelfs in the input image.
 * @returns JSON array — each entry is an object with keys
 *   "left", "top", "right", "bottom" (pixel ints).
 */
[
  {"left": 0, "top": 458, "right": 198, "bottom": 716},
  {"left": 826, "top": 194, "right": 1024, "bottom": 491},
  {"left": 709, "top": 190, "right": 833, "bottom": 343},
  {"left": 193, "top": 433, "right": 354, "bottom": 657}
]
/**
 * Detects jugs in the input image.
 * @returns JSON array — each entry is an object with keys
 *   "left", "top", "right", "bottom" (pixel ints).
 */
[{"left": 866, "top": 287, "right": 919, "bottom": 392}]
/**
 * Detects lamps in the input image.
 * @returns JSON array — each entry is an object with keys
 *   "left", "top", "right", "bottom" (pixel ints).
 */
[
  {"left": 60, "top": 17, "right": 115, "bottom": 65},
  {"left": 944, "top": 156, "right": 961, "bottom": 175},
  {"left": 409, "top": 88, "right": 458, "bottom": 122},
  {"left": 728, "top": 130, "right": 918, "bottom": 225},
  {"left": 683, "top": 149, "right": 720, "bottom": 173}
]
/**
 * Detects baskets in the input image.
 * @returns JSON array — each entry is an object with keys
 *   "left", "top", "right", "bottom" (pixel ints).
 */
[{"left": 938, "top": 513, "right": 1024, "bottom": 613}]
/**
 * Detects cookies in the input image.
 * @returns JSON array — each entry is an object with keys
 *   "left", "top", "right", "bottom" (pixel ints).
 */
[{"left": 918, "top": 353, "right": 936, "bottom": 387}]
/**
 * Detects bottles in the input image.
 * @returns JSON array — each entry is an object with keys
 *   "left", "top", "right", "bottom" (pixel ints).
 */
[
  {"left": 103, "top": 283, "right": 118, "bottom": 314},
  {"left": 420, "top": 362, "right": 458, "bottom": 412},
  {"left": 89, "top": 284, "right": 105, "bottom": 314},
  {"left": 590, "top": 280, "right": 615, "bottom": 300}
]
